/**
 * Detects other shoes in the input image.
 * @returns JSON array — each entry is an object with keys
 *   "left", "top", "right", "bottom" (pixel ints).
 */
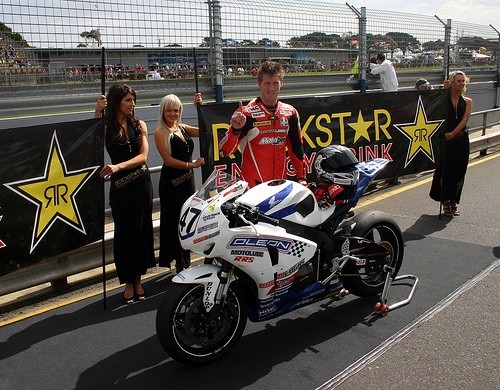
[{"left": 124, "top": 287, "right": 145, "bottom": 304}]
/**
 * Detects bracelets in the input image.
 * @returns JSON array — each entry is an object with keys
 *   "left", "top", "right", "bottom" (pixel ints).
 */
[{"left": 184, "top": 162, "right": 190, "bottom": 169}]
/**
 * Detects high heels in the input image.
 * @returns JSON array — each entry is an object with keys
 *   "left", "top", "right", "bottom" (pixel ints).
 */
[{"left": 444, "top": 206, "right": 460, "bottom": 216}]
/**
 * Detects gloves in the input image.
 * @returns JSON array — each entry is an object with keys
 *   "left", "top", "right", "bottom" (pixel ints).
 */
[{"left": 306, "top": 181, "right": 344, "bottom": 210}]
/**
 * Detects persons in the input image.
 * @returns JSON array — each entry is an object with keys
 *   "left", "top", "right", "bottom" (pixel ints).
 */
[
  {"left": 43, "top": 53, "right": 449, "bottom": 82},
  {"left": 152, "top": 92, "right": 205, "bottom": 276},
  {"left": 413, "top": 77, "right": 434, "bottom": 90},
  {"left": 96, "top": 83, "right": 153, "bottom": 304},
  {"left": 367, "top": 52, "right": 399, "bottom": 92},
  {"left": 429, "top": 72, "right": 474, "bottom": 218},
  {"left": 0, "top": 44, "right": 33, "bottom": 69},
  {"left": 219, "top": 61, "right": 308, "bottom": 192}
]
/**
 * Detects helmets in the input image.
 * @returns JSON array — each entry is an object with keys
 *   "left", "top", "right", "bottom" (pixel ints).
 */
[{"left": 314, "top": 145, "right": 359, "bottom": 185}]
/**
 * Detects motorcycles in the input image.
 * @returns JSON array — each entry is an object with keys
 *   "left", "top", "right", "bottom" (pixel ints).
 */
[{"left": 155, "top": 157, "right": 419, "bottom": 367}]
